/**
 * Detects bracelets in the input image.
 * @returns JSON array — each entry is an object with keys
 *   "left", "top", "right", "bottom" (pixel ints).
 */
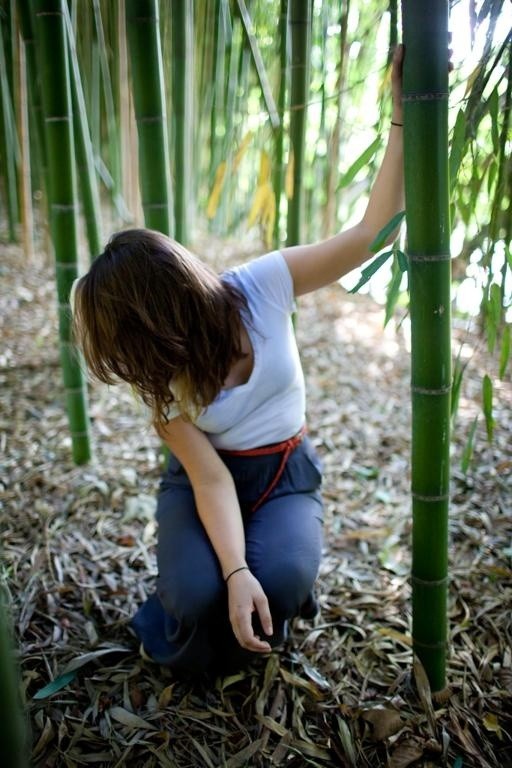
[
  {"left": 391, "top": 121, "right": 404, "bottom": 127},
  {"left": 225, "top": 567, "right": 249, "bottom": 584}
]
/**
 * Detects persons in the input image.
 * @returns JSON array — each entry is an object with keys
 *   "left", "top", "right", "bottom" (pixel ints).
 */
[{"left": 70, "top": 30, "right": 455, "bottom": 668}]
